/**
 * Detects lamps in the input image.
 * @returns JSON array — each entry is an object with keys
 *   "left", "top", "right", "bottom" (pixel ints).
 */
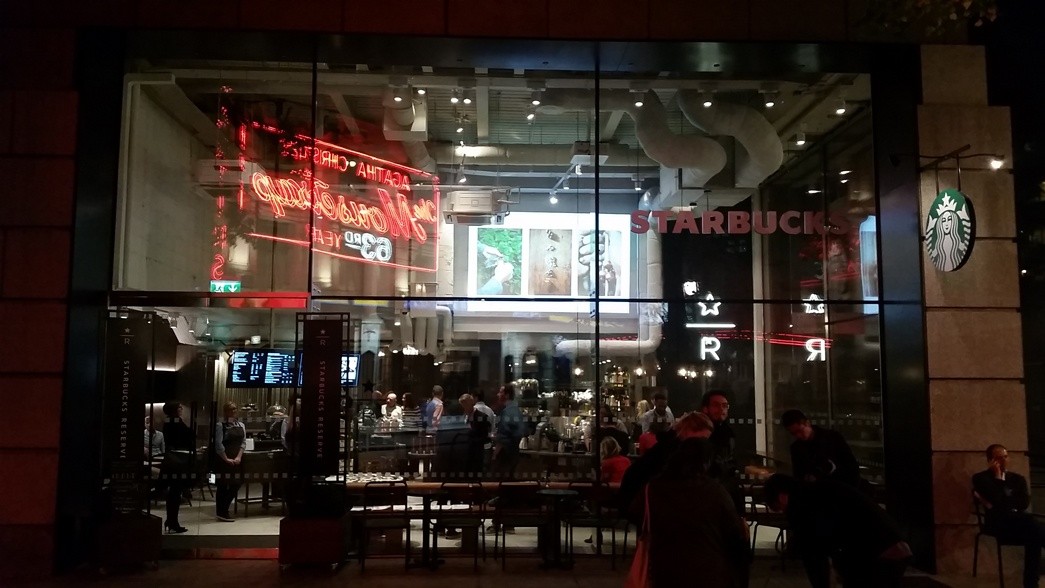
[
  {"left": 393, "top": 89, "right": 403, "bottom": 102},
  {"left": 633, "top": 92, "right": 645, "bottom": 107},
  {"left": 525, "top": 351, "right": 537, "bottom": 364},
  {"left": 634, "top": 181, "right": 641, "bottom": 191},
  {"left": 703, "top": 94, "right": 712, "bottom": 108},
  {"left": 458, "top": 167, "right": 466, "bottom": 182},
  {"left": 463, "top": 89, "right": 472, "bottom": 104},
  {"left": 575, "top": 165, "right": 584, "bottom": 175},
  {"left": 796, "top": 132, "right": 807, "bottom": 145},
  {"left": 450, "top": 88, "right": 458, "bottom": 103},
  {"left": 765, "top": 95, "right": 775, "bottom": 107},
  {"left": 417, "top": 88, "right": 426, "bottom": 95},
  {"left": 455, "top": 125, "right": 464, "bottom": 134},
  {"left": 563, "top": 179, "right": 569, "bottom": 190},
  {"left": 531, "top": 92, "right": 541, "bottom": 106}
]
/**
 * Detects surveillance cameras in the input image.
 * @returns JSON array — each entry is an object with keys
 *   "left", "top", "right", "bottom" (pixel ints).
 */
[{"left": 401, "top": 309, "right": 408, "bottom": 315}]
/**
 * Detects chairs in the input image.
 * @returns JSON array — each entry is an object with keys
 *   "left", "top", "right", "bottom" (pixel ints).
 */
[
  {"left": 360, "top": 480, "right": 411, "bottom": 571},
  {"left": 972, "top": 491, "right": 1045, "bottom": 588},
  {"left": 494, "top": 479, "right": 554, "bottom": 572},
  {"left": 564, "top": 480, "right": 619, "bottom": 572},
  {"left": 153, "top": 448, "right": 215, "bottom": 508},
  {"left": 432, "top": 477, "right": 487, "bottom": 571},
  {"left": 736, "top": 479, "right": 790, "bottom": 570}
]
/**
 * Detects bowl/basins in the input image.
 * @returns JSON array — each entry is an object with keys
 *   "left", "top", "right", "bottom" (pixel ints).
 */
[{"left": 249, "top": 404, "right": 256, "bottom": 407}]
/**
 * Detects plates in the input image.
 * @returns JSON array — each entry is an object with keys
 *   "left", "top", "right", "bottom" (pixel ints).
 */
[{"left": 241, "top": 409, "right": 251, "bottom": 411}]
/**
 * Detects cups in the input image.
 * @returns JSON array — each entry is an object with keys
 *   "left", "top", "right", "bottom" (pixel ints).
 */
[
  {"left": 585, "top": 403, "right": 591, "bottom": 414},
  {"left": 634, "top": 443, "right": 639, "bottom": 455},
  {"left": 558, "top": 441, "right": 564, "bottom": 453}
]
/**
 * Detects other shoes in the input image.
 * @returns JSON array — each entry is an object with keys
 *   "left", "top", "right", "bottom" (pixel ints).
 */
[
  {"left": 502, "top": 526, "right": 515, "bottom": 534},
  {"left": 438, "top": 530, "right": 446, "bottom": 535},
  {"left": 446, "top": 531, "right": 464, "bottom": 538},
  {"left": 216, "top": 513, "right": 235, "bottom": 521},
  {"left": 480, "top": 526, "right": 503, "bottom": 535}
]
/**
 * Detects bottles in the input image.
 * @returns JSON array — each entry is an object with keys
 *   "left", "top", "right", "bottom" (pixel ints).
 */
[
  {"left": 366, "top": 462, "right": 372, "bottom": 473},
  {"left": 630, "top": 443, "right": 634, "bottom": 455},
  {"left": 602, "top": 388, "right": 630, "bottom": 406},
  {"left": 528, "top": 435, "right": 537, "bottom": 449},
  {"left": 562, "top": 417, "right": 590, "bottom": 439},
  {"left": 519, "top": 438, "right": 527, "bottom": 450},
  {"left": 604, "top": 366, "right": 624, "bottom": 387}
]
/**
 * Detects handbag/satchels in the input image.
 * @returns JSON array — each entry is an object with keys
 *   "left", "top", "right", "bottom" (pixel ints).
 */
[{"left": 622, "top": 484, "right": 652, "bottom": 587}]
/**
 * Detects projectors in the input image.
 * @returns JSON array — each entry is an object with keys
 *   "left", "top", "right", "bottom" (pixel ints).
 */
[{"left": 444, "top": 190, "right": 500, "bottom": 224}]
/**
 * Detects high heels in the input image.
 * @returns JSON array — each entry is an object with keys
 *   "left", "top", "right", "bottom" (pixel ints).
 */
[{"left": 164, "top": 520, "right": 188, "bottom": 533}]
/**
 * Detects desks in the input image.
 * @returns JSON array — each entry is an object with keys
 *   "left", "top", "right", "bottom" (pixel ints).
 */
[
  {"left": 522, "top": 449, "right": 596, "bottom": 479},
  {"left": 533, "top": 488, "right": 576, "bottom": 572},
  {"left": 237, "top": 452, "right": 286, "bottom": 517},
  {"left": 408, "top": 488, "right": 444, "bottom": 570}
]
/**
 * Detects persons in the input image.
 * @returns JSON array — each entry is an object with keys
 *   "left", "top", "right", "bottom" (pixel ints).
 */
[
  {"left": 214, "top": 400, "right": 246, "bottom": 521},
  {"left": 144, "top": 416, "right": 165, "bottom": 478},
  {"left": 162, "top": 397, "right": 206, "bottom": 533},
  {"left": 261, "top": 384, "right": 911, "bottom": 588},
  {"left": 971, "top": 444, "right": 1045, "bottom": 588}
]
